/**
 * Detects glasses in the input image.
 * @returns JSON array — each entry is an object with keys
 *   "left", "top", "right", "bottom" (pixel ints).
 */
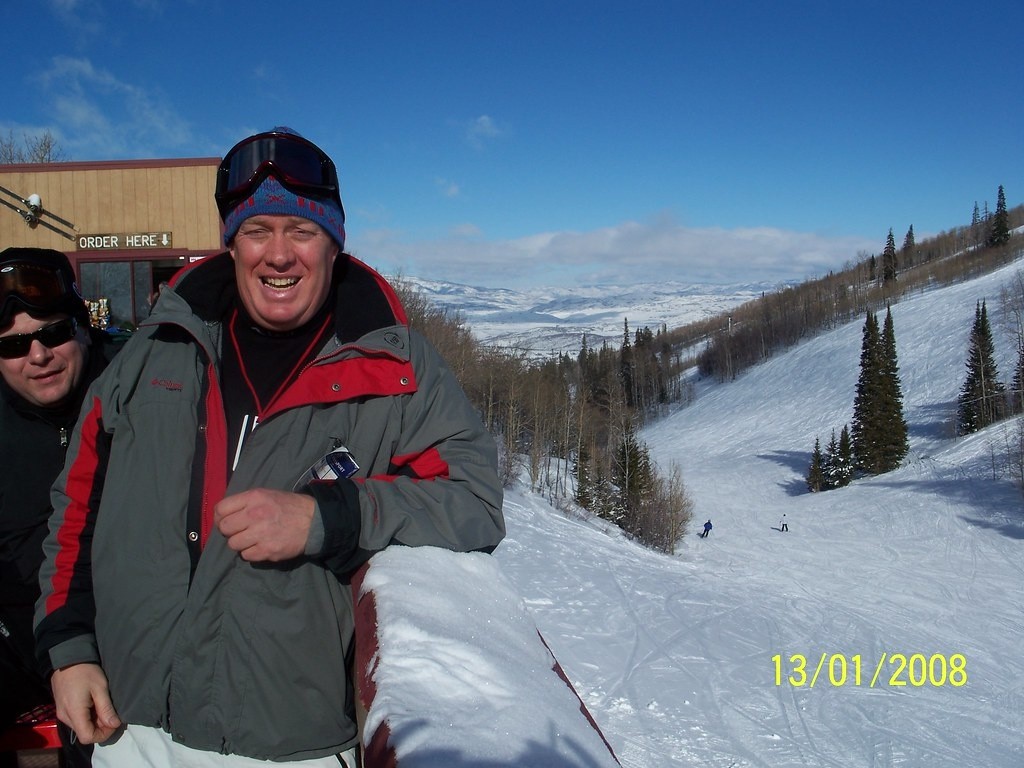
[
  {"left": 0, "top": 260, "right": 71, "bottom": 330},
  {"left": 214, "top": 132, "right": 346, "bottom": 225},
  {"left": 0, "top": 316, "right": 78, "bottom": 359}
]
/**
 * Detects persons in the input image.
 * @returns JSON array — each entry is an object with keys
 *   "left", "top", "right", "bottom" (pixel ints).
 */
[
  {"left": 702, "top": 519, "right": 712, "bottom": 538},
  {"left": 780, "top": 514, "right": 789, "bottom": 531},
  {"left": 1, "top": 247, "right": 130, "bottom": 768},
  {"left": 31, "top": 125, "right": 507, "bottom": 768}
]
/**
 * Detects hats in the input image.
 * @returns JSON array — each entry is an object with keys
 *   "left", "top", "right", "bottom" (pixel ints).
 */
[
  {"left": 0, "top": 249, "right": 90, "bottom": 328},
  {"left": 224, "top": 128, "right": 345, "bottom": 252}
]
